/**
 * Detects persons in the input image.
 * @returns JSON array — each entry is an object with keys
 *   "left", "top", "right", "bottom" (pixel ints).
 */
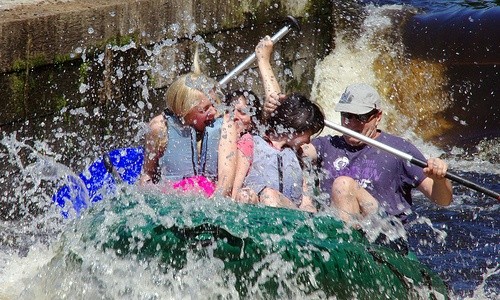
[
  {"left": 139, "top": 35, "right": 325, "bottom": 213},
  {"left": 262, "top": 84, "right": 454, "bottom": 225}
]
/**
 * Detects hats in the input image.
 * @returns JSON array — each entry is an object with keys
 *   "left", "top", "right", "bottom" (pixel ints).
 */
[{"left": 334, "top": 83, "right": 382, "bottom": 115}]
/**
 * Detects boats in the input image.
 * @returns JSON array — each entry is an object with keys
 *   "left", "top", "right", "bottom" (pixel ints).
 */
[{"left": 65, "top": 190, "right": 451, "bottom": 300}]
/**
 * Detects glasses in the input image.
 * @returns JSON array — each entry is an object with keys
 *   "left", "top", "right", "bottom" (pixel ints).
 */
[{"left": 341, "top": 108, "right": 377, "bottom": 121}]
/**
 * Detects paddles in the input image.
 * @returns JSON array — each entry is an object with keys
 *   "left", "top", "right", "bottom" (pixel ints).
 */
[
  {"left": 51, "top": 15, "right": 301, "bottom": 221},
  {"left": 320, "top": 118, "right": 500, "bottom": 202}
]
